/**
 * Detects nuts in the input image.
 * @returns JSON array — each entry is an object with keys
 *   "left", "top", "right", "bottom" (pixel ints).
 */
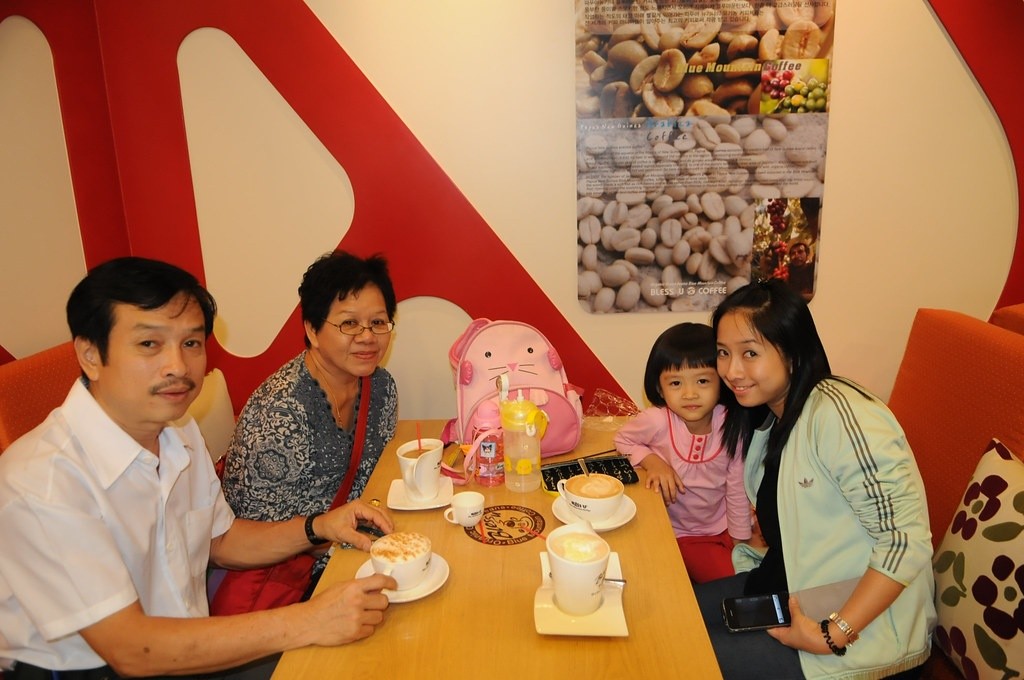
[{"left": 575, "top": 0, "right": 837, "bottom": 313}]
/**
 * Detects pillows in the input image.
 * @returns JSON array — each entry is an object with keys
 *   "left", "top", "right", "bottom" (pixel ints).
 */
[
  {"left": 928, "top": 437, "right": 1024, "bottom": 680},
  {"left": 180, "top": 365, "right": 237, "bottom": 460}
]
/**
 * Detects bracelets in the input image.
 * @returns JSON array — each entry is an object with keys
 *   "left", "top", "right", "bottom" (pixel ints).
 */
[
  {"left": 829, "top": 612, "right": 859, "bottom": 646},
  {"left": 304, "top": 512, "right": 328, "bottom": 546},
  {"left": 821, "top": 619, "right": 846, "bottom": 656}
]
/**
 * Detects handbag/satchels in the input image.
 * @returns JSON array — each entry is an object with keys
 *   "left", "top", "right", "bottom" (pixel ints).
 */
[{"left": 208, "top": 554, "right": 317, "bottom": 617}]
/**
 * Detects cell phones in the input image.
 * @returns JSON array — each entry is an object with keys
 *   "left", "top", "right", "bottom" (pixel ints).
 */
[{"left": 722, "top": 592, "right": 791, "bottom": 632}]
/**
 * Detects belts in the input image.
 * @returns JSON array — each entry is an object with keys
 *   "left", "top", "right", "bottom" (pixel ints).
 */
[{"left": 14, "top": 662, "right": 118, "bottom": 680}]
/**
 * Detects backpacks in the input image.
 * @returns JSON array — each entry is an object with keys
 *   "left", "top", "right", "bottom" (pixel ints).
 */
[{"left": 441, "top": 317, "right": 584, "bottom": 461}]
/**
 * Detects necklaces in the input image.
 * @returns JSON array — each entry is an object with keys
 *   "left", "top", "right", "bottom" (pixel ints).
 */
[{"left": 310, "top": 353, "right": 345, "bottom": 429}]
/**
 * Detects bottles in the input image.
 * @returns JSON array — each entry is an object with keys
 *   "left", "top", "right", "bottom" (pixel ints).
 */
[
  {"left": 472, "top": 399, "right": 504, "bottom": 487},
  {"left": 500, "top": 389, "right": 546, "bottom": 493}
]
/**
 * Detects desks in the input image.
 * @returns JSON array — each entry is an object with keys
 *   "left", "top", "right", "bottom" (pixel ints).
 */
[{"left": 268, "top": 415, "right": 724, "bottom": 680}]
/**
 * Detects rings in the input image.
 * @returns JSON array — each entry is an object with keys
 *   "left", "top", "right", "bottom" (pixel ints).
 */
[{"left": 369, "top": 498, "right": 381, "bottom": 508}]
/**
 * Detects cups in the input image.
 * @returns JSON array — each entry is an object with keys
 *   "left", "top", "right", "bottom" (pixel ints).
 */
[
  {"left": 396, "top": 439, "right": 444, "bottom": 502},
  {"left": 546, "top": 522, "right": 610, "bottom": 614},
  {"left": 370, "top": 532, "right": 432, "bottom": 590},
  {"left": 557, "top": 473, "right": 624, "bottom": 522},
  {"left": 443, "top": 492, "right": 485, "bottom": 527}
]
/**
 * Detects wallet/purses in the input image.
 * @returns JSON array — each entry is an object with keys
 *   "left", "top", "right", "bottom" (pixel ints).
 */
[{"left": 539, "top": 456, "right": 641, "bottom": 491}]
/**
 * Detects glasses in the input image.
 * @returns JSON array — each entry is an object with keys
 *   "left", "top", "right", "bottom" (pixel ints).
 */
[{"left": 324, "top": 319, "right": 395, "bottom": 335}]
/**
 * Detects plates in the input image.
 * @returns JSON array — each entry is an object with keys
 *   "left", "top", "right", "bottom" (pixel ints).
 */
[
  {"left": 355, "top": 552, "right": 449, "bottom": 603},
  {"left": 534, "top": 552, "right": 630, "bottom": 636},
  {"left": 552, "top": 495, "right": 636, "bottom": 531},
  {"left": 387, "top": 476, "right": 454, "bottom": 509}
]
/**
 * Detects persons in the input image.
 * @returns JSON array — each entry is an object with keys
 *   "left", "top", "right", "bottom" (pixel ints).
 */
[
  {"left": 205, "top": 247, "right": 397, "bottom": 616},
  {"left": 692, "top": 276, "right": 939, "bottom": 680},
  {"left": 613, "top": 321, "right": 764, "bottom": 584},
  {"left": 0, "top": 255, "right": 398, "bottom": 679}
]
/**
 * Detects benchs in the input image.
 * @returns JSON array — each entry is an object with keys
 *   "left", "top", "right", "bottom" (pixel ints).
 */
[
  {"left": 885, "top": 311, "right": 1024, "bottom": 680},
  {"left": 0, "top": 333, "right": 238, "bottom": 486}
]
